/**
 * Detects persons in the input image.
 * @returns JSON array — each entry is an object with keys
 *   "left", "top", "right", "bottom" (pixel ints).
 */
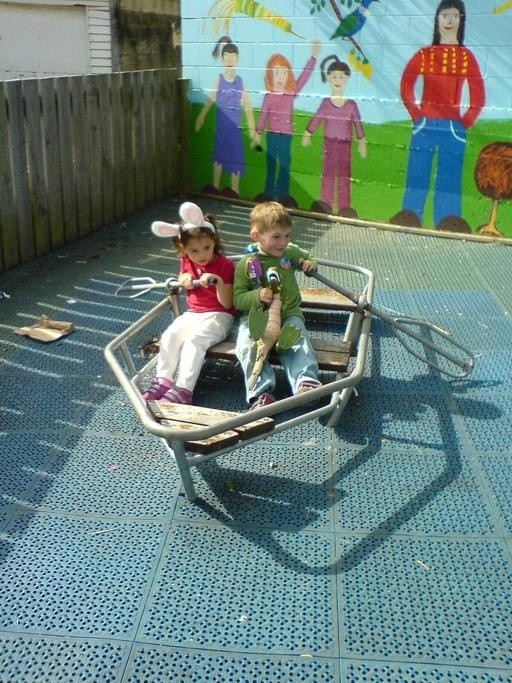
[
  {"left": 231, "top": 198, "right": 322, "bottom": 415},
  {"left": 141, "top": 198, "right": 235, "bottom": 408},
  {"left": 301, "top": 54, "right": 369, "bottom": 221},
  {"left": 389, "top": 1, "right": 487, "bottom": 234},
  {"left": 256, "top": 39, "right": 324, "bottom": 209},
  {"left": 196, "top": 35, "right": 257, "bottom": 200}
]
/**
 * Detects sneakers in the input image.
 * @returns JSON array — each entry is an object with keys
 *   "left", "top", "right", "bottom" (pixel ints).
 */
[
  {"left": 297, "top": 381, "right": 321, "bottom": 405},
  {"left": 249, "top": 392, "right": 277, "bottom": 410},
  {"left": 143, "top": 377, "right": 171, "bottom": 400},
  {"left": 159, "top": 386, "right": 194, "bottom": 404}
]
